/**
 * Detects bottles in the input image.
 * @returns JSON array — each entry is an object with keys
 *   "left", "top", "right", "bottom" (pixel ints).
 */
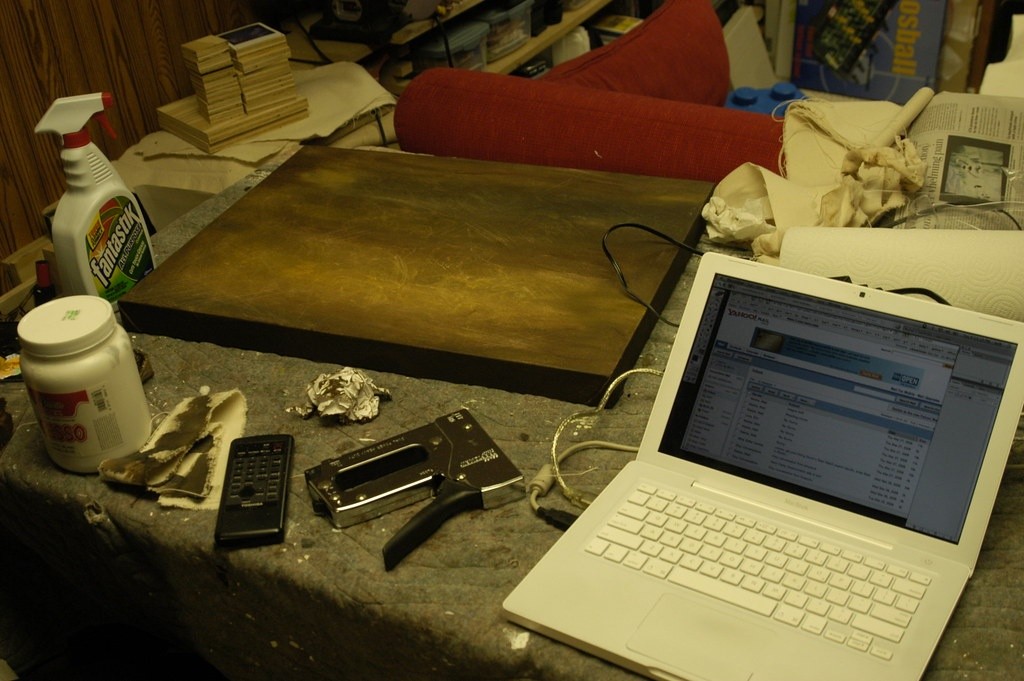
[{"left": 17, "top": 295, "right": 153, "bottom": 474}]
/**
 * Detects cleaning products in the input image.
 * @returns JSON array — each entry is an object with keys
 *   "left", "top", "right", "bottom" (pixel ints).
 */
[{"left": 33, "top": 91, "right": 158, "bottom": 312}]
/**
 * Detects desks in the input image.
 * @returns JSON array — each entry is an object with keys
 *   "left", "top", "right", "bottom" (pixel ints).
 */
[
  {"left": 0, "top": 142, "right": 1024, "bottom": 681},
  {"left": 127, "top": 61, "right": 396, "bottom": 170}
]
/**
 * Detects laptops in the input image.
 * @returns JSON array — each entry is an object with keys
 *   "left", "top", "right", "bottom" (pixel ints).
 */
[{"left": 503, "top": 251, "right": 1024, "bottom": 681}]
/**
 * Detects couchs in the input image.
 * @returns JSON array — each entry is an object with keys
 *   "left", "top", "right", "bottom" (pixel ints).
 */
[{"left": 394, "top": 0, "right": 787, "bottom": 180}]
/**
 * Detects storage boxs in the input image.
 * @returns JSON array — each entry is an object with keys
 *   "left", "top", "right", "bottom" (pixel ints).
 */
[
  {"left": 480, "top": 0, "right": 534, "bottom": 63},
  {"left": 424, "top": 22, "right": 489, "bottom": 74}
]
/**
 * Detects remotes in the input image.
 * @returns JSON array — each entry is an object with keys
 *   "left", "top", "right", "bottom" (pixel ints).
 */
[{"left": 211, "top": 434, "right": 294, "bottom": 548}]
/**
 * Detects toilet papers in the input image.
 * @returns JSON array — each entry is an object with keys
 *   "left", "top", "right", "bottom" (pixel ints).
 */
[{"left": 778, "top": 228, "right": 1024, "bottom": 323}]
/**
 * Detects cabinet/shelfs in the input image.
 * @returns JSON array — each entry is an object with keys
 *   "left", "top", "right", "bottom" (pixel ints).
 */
[{"left": 308, "top": 0, "right": 609, "bottom": 96}]
